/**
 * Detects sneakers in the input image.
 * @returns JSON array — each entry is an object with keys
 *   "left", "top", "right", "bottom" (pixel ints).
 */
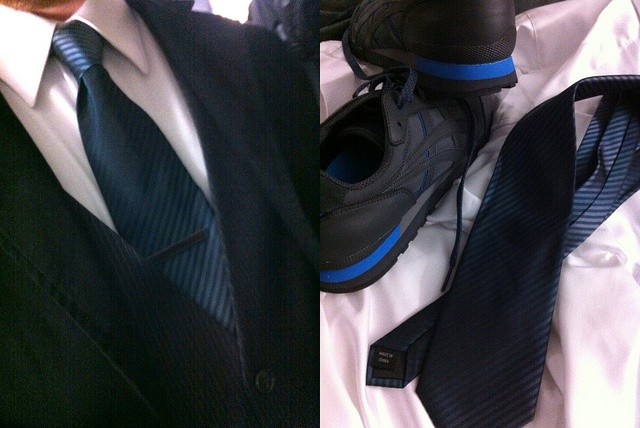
[
  {"left": 321, "top": 64, "right": 499, "bottom": 293},
  {"left": 347, "top": 0, "right": 518, "bottom": 99}
]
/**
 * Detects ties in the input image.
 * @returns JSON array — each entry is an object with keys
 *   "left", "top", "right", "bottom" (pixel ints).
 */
[
  {"left": 50, "top": 20, "right": 239, "bottom": 341},
  {"left": 366, "top": 74, "right": 639, "bottom": 428}
]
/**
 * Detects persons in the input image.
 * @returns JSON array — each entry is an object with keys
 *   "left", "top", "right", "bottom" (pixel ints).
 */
[{"left": 0, "top": 0, "right": 320, "bottom": 428}]
